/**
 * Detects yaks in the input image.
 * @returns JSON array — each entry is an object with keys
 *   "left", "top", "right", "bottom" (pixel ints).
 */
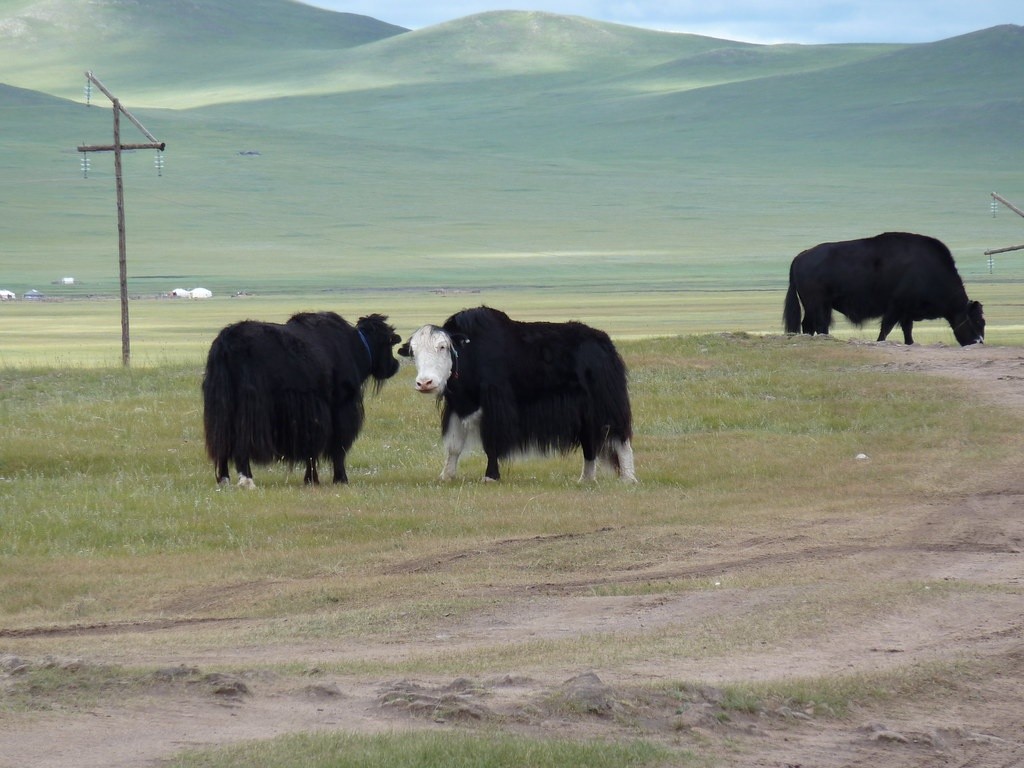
[
  {"left": 399, "top": 306, "right": 637, "bottom": 489},
  {"left": 780, "top": 232, "right": 986, "bottom": 346},
  {"left": 201, "top": 311, "right": 400, "bottom": 489}
]
